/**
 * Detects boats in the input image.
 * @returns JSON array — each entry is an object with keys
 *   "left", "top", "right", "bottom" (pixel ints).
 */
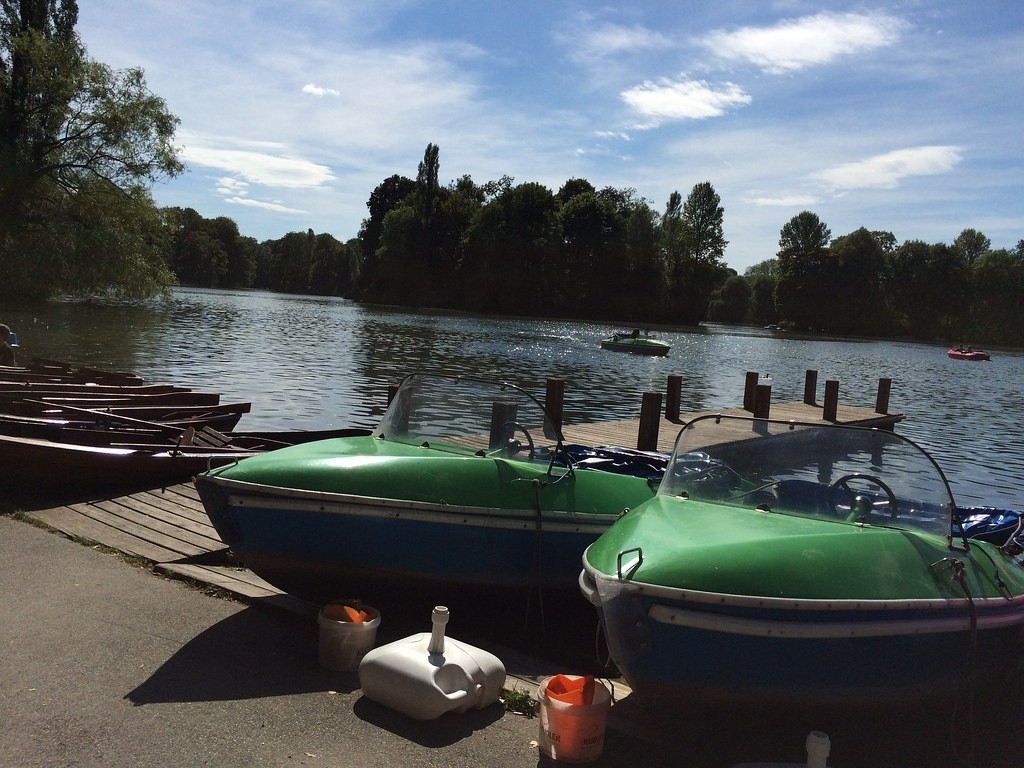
[
  {"left": 772, "top": 328, "right": 787, "bottom": 332},
  {"left": 192, "top": 374, "right": 778, "bottom": 625},
  {"left": 0, "top": 363, "right": 375, "bottom": 500},
  {"left": 947, "top": 348, "right": 991, "bottom": 361},
  {"left": 764, "top": 324, "right": 778, "bottom": 329},
  {"left": 577, "top": 413, "right": 1024, "bottom": 727},
  {"left": 601, "top": 334, "right": 671, "bottom": 357}
]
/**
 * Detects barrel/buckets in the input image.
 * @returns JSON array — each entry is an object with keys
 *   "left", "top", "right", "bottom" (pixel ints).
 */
[
  {"left": 538, "top": 675, "right": 611, "bottom": 766},
  {"left": 318, "top": 601, "right": 380, "bottom": 673}
]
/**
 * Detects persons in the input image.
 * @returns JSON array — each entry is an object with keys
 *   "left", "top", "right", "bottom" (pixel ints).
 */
[
  {"left": 955, "top": 344, "right": 964, "bottom": 352},
  {"left": 631, "top": 329, "right": 640, "bottom": 338},
  {"left": 965, "top": 345, "right": 972, "bottom": 352},
  {"left": 0, "top": 324, "right": 16, "bottom": 366}
]
